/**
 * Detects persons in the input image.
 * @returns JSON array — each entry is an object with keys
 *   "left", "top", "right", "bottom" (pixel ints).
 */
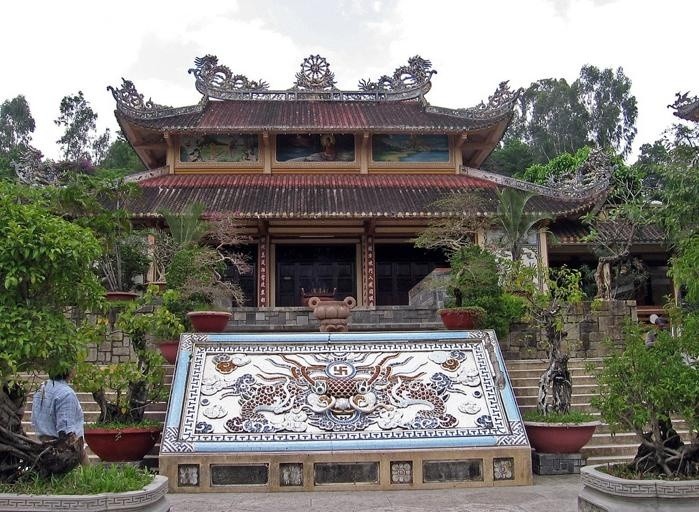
[
  {"left": 30, "top": 362, "right": 92, "bottom": 468},
  {"left": 645, "top": 317, "right": 673, "bottom": 348}
]
